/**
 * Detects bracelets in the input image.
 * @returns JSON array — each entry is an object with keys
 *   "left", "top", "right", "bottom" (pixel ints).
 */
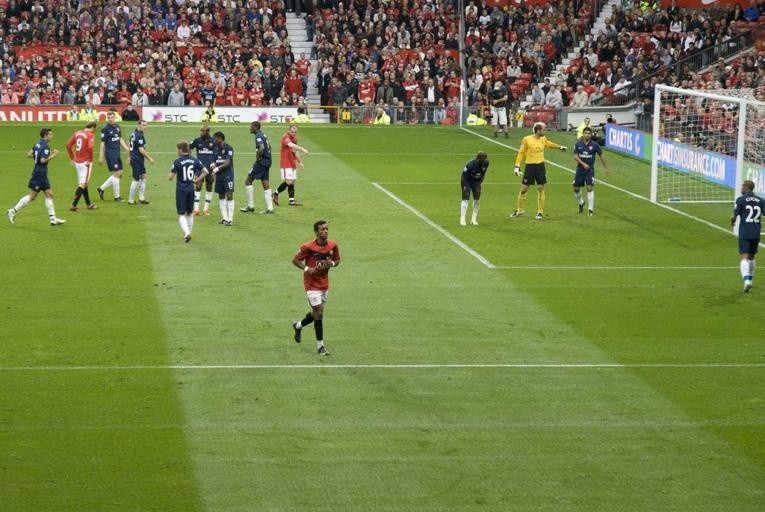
[
  {"left": 304, "top": 266, "right": 308, "bottom": 273},
  {"left": 213, "top": 167, "right": 219, "bottom": 173},
  {"left": 211, "top": 163, "right": 215, "bottom": 168},
  {"left": 332, "top": 260, "right": 335, "bottom": 266}
]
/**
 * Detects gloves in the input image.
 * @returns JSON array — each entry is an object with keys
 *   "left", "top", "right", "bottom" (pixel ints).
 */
[
  {"left": 513, "top": 167, "right": 523, "bottom": 177},
  {"left": 560, "top": 145, "right": 569, "bottom": 153}
]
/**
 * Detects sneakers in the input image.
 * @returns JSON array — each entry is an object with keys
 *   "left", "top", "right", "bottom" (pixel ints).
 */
[
  {"left": 183, "top": 234, "right": 191, "bottom": 243},
  {"left": 317, "top": 346, "right": 330, "bottom": 356},
  {"left": 5, "top": 208, "right": 17, "bottom": 225},
  {"left": 69, "top": 186, "right": 150, "bottom": 212},
  {"left": 578, "top": 200, "right": 595, "bottom": 217},
  {"left": 292, "top": 322, "right": 302, "bottom": 343},
  {"left": 535, "top": 213, "right": 543, "bottom": 219},
  {"left": 49, "top": 215, "right": 67, "bottom": 227},
  {"left": 193, "top": 192, "right": 304, "bottom": 226},
  {"left": 460, "top": 218, "right": 479, "bottom": 227},
  {"left": 742, "top": 280, "right": 754, "bottom": 294},
  {"left": 510, "top": 210, "right": 525, "bottom": 218}
]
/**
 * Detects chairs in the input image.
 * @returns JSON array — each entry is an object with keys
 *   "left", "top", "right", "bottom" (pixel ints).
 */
[
  {"left": 508, "top": 72, "right": 532, "bottom": 100},
  {"left": 525, "top": 104, "right": 555, "bottom": 128}
]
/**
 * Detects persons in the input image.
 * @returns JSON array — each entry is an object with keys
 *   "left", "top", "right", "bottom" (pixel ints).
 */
[
  {"left": 292, "top": 220, "right": 340, "bottom": 356},
  {"left": 66, "top": 121, "right": 98, "bottom": 212},
  {"left": 189, "top": 125, "right": 216, "bottom": 216},
  {"left": 271, "top": 125, "right": 309, "bottom": 205},
  {"left": 511, "top": 122, "right": 568, "bottom": 218},
  {"left": 6, "top": 127, "right": 66, "bottom": 225},
  {"left": 126, "top": 120, "right": 153, "bottom": 204},
  {"left": 459, "top": 152, "right": 490, "bottom": 226},
  {"left": 730, "top": 179, "right": 765, "bottom": 293},
  {"left": 167, "top": 142, "right": 209, "bottom": 243},
  {"left": 210, "top": 131, "right": 234, "bottom": 225},
  {"left": 96, "top": 112, "right": 129, "bottom": 202},
  {"left": 240, "top": 120, "right": 274, "bottom": 214},
  {"left": 1, "top": 0, "right": 763, "bottom": 158},
  {"left": 573, "top": 127, "right": 609, "bottom": 217}
]
